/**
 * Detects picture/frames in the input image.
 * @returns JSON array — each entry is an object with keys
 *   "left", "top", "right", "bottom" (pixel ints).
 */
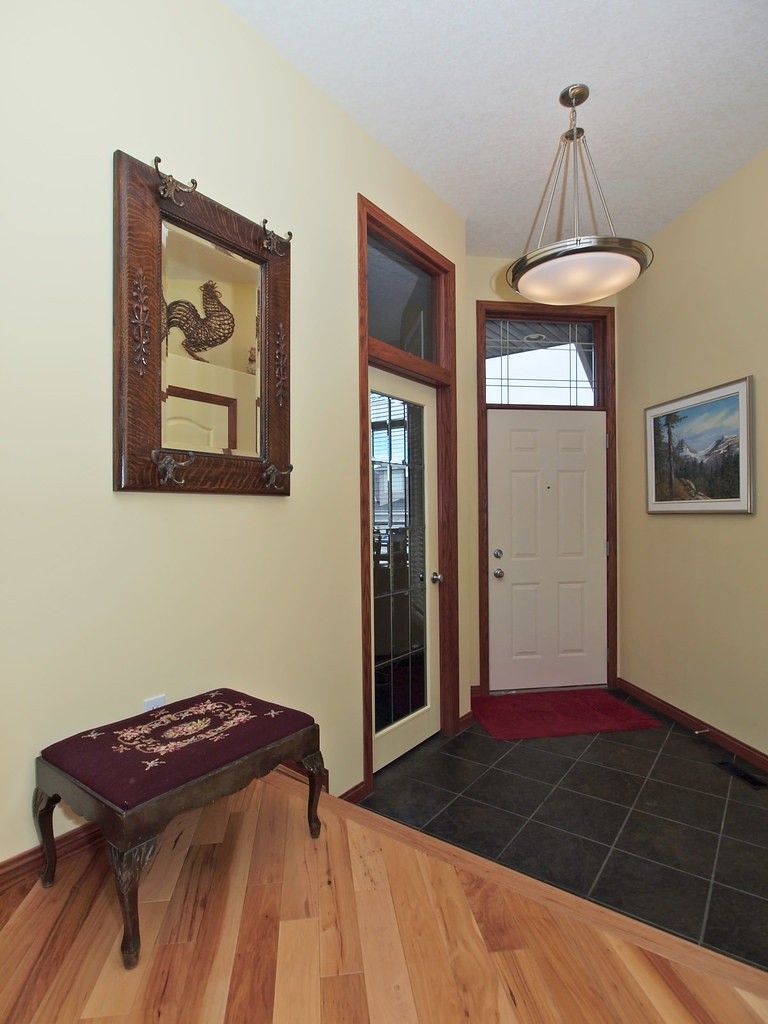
[
  {"left": 643, "top": 375, "right": 754, "bottom": 515},
  {"left": 113, "top": 150, "right": 293, "bottom": 496}
]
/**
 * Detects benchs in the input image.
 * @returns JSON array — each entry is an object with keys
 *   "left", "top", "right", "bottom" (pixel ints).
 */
[{"left": 31, "top": 688, "right": 324, "bottom": 970}]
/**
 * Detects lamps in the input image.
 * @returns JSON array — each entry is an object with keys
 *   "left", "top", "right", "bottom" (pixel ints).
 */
[{"left": 505, "top": 84, "right": 654, "bottom": 305}]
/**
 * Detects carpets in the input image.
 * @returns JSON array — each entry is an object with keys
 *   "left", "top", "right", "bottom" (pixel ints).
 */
[{"left": 471, "top": 687, "right": 664, "bottom": 741}]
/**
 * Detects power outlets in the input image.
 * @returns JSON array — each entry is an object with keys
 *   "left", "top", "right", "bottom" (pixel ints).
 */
[{"left": 144, "top": 694, "right": 165, "bottom": 713}]
